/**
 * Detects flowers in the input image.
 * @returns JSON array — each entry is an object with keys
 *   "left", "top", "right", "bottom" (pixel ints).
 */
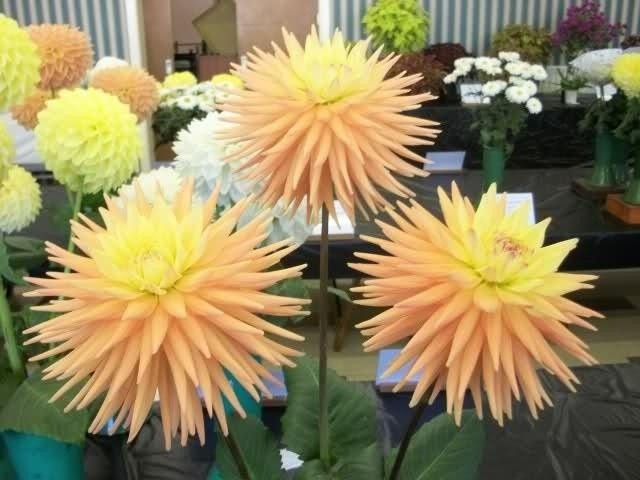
[
  {"left": 219, "top": 22, "right": 445, "bottom": 463},
  {"left": 25, "top": 177, "right": 308, "bottom": 470},
  {"left": 1, "top": 0, "right": 221, "bottom": 246},
  {"left": 371, "top": 2, "right": 636, "bottom": 206},
  {"left": 342, "top": 178, "right": 606, "bottom": 417}
]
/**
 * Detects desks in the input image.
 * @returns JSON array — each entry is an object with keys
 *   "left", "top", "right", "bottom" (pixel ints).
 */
[{"left": 286, "top": 168, "right": 640, "bottom": 354}]
